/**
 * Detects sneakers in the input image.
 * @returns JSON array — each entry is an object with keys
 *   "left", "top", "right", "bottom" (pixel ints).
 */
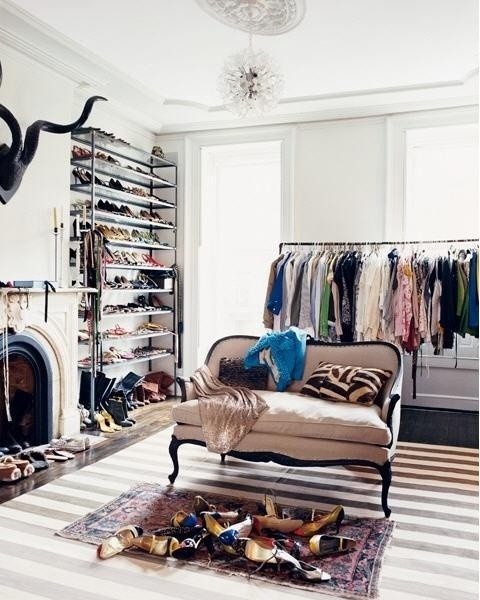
[
  {"left": 50, "top": 437, "right": 87, "bottom": 451},
  {"left": 59, "top": 434, "right": 90, "bottom": 449}
]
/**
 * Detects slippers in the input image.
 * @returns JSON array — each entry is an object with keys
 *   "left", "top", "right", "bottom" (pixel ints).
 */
[
  {"left": 40, "top": 447, "right": 68, "bottom": 463},
  {"left": 0, "top": 461, "right": 22, "bottom": 484},
  {"left": 15, "top": 450, "right": 42, "bottom": 473},
  {"left": 0, "top": 453, "right": 32, "bottom": 482},
  {"left": 27, "top": 449, "right": 49, "bottom": 470},
  {"left": 47, "top": 444, "right": 76, "bottom": 460}
]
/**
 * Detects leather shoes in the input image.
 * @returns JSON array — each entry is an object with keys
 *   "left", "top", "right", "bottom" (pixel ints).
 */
[
  {"left": 77, "top": 126, "right": 174, "bottom": 433},
  {"left": 0, "top": 429, "right": 30, "bottom": 455}
]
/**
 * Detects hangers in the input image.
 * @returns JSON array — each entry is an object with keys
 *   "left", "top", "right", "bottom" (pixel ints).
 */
[{"left": 281, "top": 238, "right": 480, "bottom": 264}]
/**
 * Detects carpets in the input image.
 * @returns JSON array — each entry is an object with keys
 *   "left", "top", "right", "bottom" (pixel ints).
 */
[
  {"left": 0, "top": 423, "right": 479, "bottom": 600},
  {"left": 55, "top": 480, "right": 398, "bottom": 600}
]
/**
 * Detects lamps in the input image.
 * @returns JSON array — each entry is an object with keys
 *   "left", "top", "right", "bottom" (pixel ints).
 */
[{"left": 199, "top": 0, "right": 307, "bottom": 120}]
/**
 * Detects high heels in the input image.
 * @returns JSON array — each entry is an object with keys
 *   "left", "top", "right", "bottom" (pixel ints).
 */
[{"left": 95, "top": 488, "right": 357, "bottom": 585}]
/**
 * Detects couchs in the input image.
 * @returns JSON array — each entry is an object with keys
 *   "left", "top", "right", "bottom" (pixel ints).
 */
[{"left": 167, "top": 334, "right": 403, "bottom": 519}]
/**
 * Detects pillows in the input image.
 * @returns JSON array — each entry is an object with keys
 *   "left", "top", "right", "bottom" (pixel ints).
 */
[{"left": 300, "top": 361, "right": 393, "bottom": 406}]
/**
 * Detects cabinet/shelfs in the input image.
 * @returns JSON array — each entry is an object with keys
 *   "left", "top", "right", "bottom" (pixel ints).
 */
[{"left": 70, "top": 128, "right": 178, "bottom": 431}]
